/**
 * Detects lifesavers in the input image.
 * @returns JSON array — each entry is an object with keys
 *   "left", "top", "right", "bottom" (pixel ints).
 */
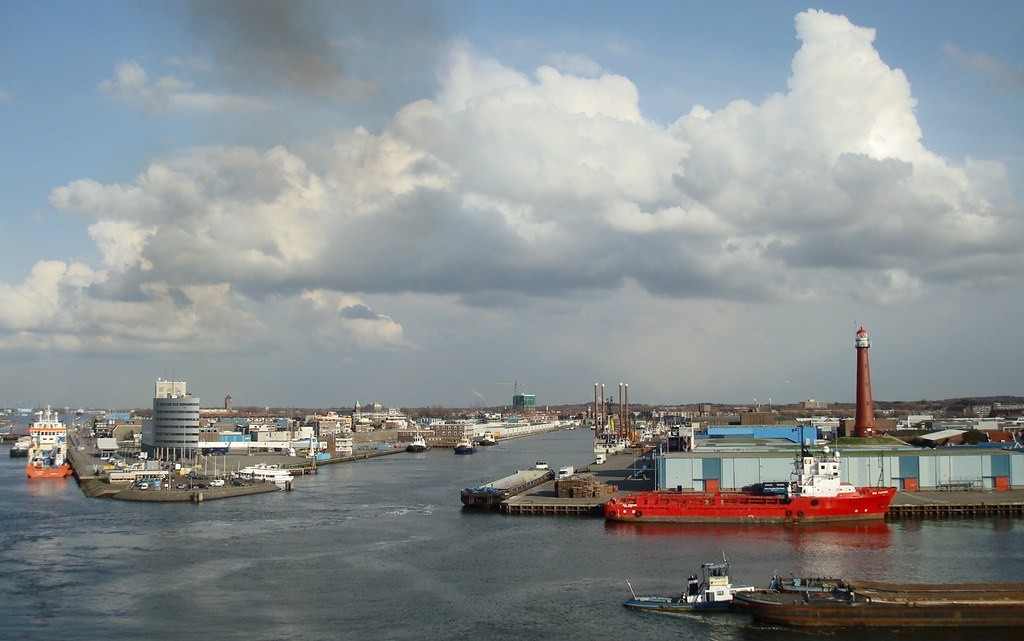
[
  {"left": 812, "top": 500, "right": 819, "bottom": 506},
  {"left": 634, "top": 511, "right": 642, "bottom": 517},
  {"left": 609, "top": 511, "right": 615, "bottom": 517}
]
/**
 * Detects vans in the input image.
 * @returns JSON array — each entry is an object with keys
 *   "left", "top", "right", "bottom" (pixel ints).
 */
[{"left": 209, "top": 480, "right": 224, "bottom": 487}]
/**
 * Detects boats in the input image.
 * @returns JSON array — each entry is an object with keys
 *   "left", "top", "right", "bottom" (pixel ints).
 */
[
  {"left": 479, "top": 431, "right": 498, "bottom": 445},
  {"left": 732, "top": 577, "right": 1024, "bottom": 636},
  {"left": 604, "top": 427, "right": 897, "bottom": 522},
  {"left": 622, "top": 556, "right": 753, "bottom": 611},
  {"left": 26, "top": 406, "right": 72, "bottom": 477},
  {"left": 10, "top": 436, "right": 28, "bottom": 456},
  {"left": 406, "top": 433, "right": 426, "bottom": 453},
  {"left": 604, "top": 521, "right": 895, "bottom": 548},
  {"left": 238, "top": 462, "right": 295, "bottom": 491},
  {"left": 461, "top": 457, "right": 556, "bottom": 508},
  {"left": 453, "top": 435, "right": 476, "bottom": 454}
]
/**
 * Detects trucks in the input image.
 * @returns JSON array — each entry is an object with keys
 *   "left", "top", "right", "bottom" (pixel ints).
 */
[{"left": 560, "top": 465, "right": 574, "bottom": 478}]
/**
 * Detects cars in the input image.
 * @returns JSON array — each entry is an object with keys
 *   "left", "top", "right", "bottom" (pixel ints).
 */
[
  {"left": 141, "top": 483, "right": 148, "bottom": 490},
  {"left": 231, "top": 479, "right": 248, "bottom": 487},
  {"left": 187, "top": 483, "right": 208, "bottom": 489},
  {"left": 102, "top": 456, "right": 125, "bottom": 468},
  {"left": 176, "top": 483, "right": 185, "bottom": 489}
]
